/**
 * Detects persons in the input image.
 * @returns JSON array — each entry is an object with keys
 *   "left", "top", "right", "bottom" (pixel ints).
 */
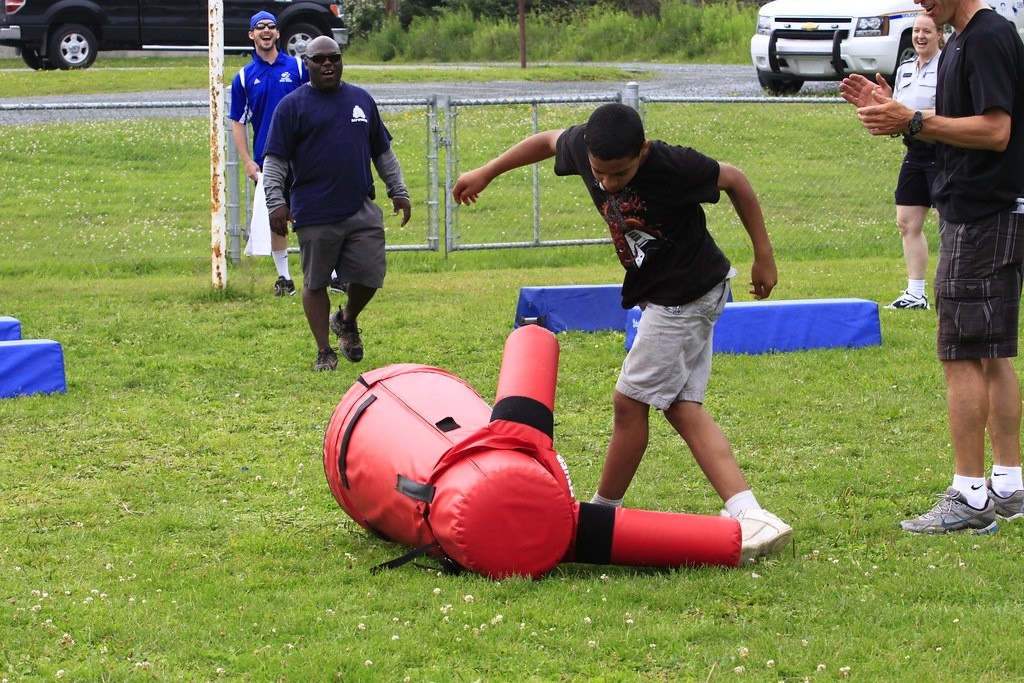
[
  {"left": 261, "top": 36, "right": 412, "bottom": 371},
  {"left": 840, "top": 0, "right": 1024, "bottom": 535},
  {"left": 230, "top": 10, "right": 348, "bottom": 299},
  {"left": 883, "top": 11, "right": 945, "bottom": 309},
  {"left": 452, "top": 104, "right": 793, "bottom": 562}
]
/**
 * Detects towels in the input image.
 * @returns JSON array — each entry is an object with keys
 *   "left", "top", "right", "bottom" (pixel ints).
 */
[{"left": 242, "top": 170, "right": 272, "bottom": 257}]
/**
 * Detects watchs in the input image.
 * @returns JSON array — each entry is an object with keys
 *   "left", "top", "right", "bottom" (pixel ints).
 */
[{"left": 909, "top": 111, "right": 922, "bottom": 136}]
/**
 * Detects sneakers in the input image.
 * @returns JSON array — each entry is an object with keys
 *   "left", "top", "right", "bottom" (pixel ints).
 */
[
  {"left": 312, "top": 346, "right": 339, "bottom": 373},
  {"left": 985, "top": 476, "right": 1024, "bottom": 523},
  {"left": 328, "top": 304, "right": 364, "bottom": 363},
  {"left": 274, "top": 276, "right": 297, "bottom": 298},
  {"left": 882, "top": 287, "right": 932, "bottom": 311},
  {"left": 897, "top": 485, "right": 999, "bottom": 537},
  {"left": 718, "top": 507, "right": 793, "bottom": 568},
  {"left": 329, "top": 277, "right": 347, "bottom": 294}
]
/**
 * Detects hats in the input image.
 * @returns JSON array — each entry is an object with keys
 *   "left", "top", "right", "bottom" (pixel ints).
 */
[{"left": 249, "top": 10, "right": 278, "bottom": 32}]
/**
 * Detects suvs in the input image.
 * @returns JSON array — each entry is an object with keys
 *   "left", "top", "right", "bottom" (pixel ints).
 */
[{"left": 750, "top": 0, "right": 1024, "bottom": 98}]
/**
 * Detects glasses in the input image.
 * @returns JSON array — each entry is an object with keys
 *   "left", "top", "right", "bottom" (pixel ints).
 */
[
  {"left": 253, "top": 22, "right": 277, "bottom": 30},
  {"left": 300, "top": 52, "right": 342, "bottom": 63}
]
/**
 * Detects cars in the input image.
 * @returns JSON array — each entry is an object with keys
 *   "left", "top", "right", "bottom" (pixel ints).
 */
[{"left": 0, "top": 0, "right": 351, "bottom": 73}]
